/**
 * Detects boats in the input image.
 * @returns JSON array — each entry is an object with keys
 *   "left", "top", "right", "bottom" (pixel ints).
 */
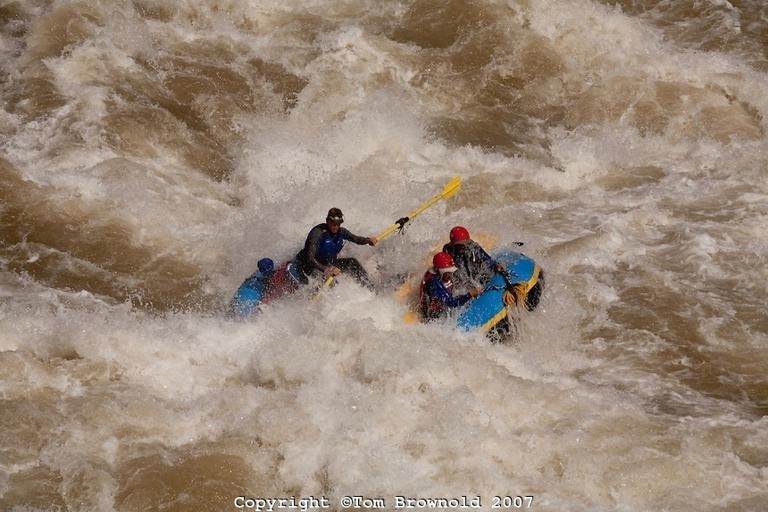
[{"left": 224, "top": 247, "right": 544, "bottom": 344}]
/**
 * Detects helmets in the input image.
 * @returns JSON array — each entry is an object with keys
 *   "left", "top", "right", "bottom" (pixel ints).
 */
[
  {"left": 449, "top": 226, "right": 470, "bottom": 245},
  {"left": 433, "top": 253, "right": 458, "bottom": 274}
]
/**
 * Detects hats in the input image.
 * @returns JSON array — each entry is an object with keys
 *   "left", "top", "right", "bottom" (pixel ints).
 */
[{"left": 326, "top": 207, "right": 345, "bottom": 225}]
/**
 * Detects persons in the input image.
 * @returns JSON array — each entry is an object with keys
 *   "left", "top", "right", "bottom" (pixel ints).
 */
[
  {"left": 417, "top": 252, "right": 482, "bottom": 326},
  {"left": 297, "top": 206, "right": 380, "bottom": 296},
  {"left": 443, "top": 224, "right": 506, "bottom": 299}
]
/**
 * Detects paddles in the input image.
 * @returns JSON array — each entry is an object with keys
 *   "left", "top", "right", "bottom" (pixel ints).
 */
[{"left": 367, "top": 176, "right": 464, "bottom": 248}]
